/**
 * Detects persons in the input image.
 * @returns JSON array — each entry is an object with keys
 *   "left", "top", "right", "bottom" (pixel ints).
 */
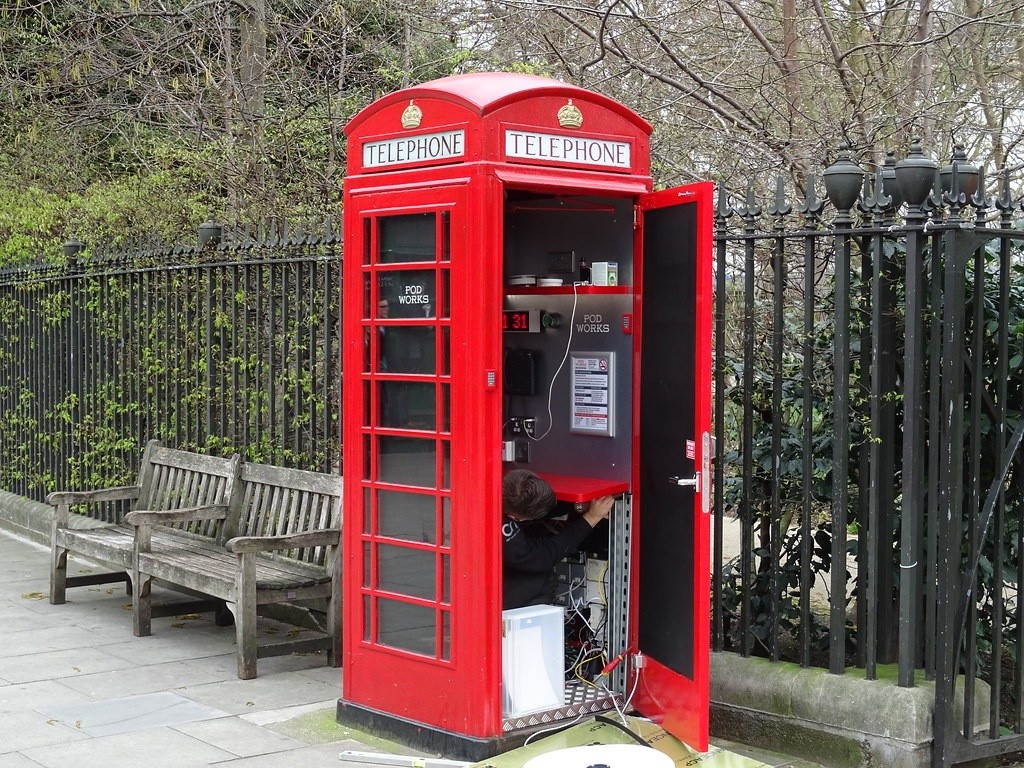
[{"left": 501, "top": 469, "right": 615, "bottom": 611}]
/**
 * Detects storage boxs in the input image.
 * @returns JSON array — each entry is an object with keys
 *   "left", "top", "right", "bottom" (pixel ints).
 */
[{"left": 591, "top": 262, "right": 618, "bottom": 287}]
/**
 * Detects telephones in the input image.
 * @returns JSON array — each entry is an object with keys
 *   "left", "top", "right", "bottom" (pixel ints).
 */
[{"left": 503, "top": 346, "right": 538, "bottom": 397}]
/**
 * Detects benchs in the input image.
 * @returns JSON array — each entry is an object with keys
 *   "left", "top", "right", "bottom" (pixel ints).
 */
[{"left": 45, "top": 438, "right": 344, "bottom": 680}]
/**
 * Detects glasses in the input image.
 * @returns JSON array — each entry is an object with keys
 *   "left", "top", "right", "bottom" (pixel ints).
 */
[{"left": 380, "top": 304, "right": 389, "bottom": 309}]
